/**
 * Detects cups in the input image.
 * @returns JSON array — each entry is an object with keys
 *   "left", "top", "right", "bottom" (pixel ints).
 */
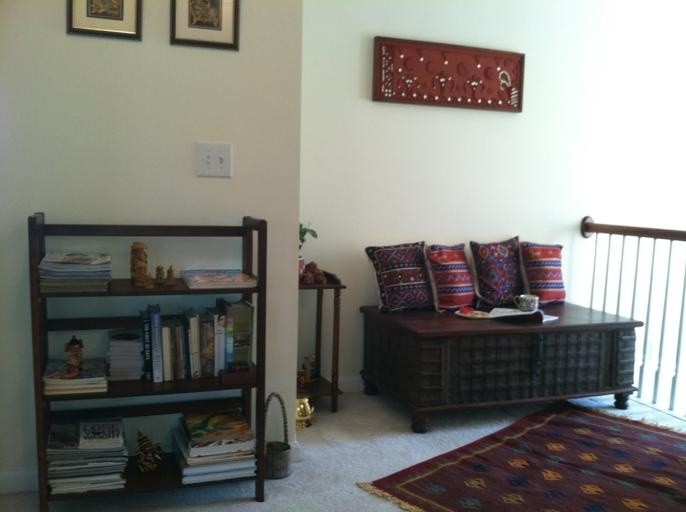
[{"left": 512, "top": 295, "right": 539, "bottom": 312}]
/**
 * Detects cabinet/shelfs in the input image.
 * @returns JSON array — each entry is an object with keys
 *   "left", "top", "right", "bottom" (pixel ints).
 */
[{"left": 25, "top": 210, "right": 270, "bottom": 512}]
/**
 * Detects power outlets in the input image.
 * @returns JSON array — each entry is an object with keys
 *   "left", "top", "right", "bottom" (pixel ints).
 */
[{"left": 193, "top": 142, "right": 232, "bottom": 179}]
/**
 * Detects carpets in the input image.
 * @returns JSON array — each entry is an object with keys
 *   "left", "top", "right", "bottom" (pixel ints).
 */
[{"left": 355, "top": 401, "right": 686, "bottom": 512}]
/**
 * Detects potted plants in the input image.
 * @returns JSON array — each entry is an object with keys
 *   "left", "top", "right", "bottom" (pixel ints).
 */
[{"left": 297, "top": 222, "right": 316, "bottom": 280}]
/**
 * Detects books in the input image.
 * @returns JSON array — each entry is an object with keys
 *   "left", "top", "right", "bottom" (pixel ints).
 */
[
  {"left": 172, "top": 409, "right": 256, "bottom": 484},
  {"left": 489, "top": 306, "right": 560, "bottom": 324},
  {"left": 38, "top": 251, "right": 112, "bottom": 293},
  {"left": 181, "top": 269, "right": 256, "bottom": 288},
  {"left": 42, "top": 299, "right": 254, "bottom": 394},
  {"left": 47, "top": 421, "right": 128, "bottom": 495}
]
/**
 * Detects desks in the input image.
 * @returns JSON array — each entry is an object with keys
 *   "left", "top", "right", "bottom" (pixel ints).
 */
[
  {"left": 359, "top": 299, "right": 644, "bottom": 436},
  {"left": 298, "top": 284, "right": 347, "bottom": 412}
]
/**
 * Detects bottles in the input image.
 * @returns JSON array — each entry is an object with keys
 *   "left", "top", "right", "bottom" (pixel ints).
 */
[{"left": 130, "top": 242, "right": 149, "bottom": 286}]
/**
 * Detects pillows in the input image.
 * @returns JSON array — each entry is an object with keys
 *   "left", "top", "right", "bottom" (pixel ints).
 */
[
  {"left": 519, "top": 239, "right": 568, "bottom": 307},
  {"left": 423, "top": 242, "right": 475, "bottom": 314},
  {"left": 468, "top": 234, "right": 527, "bottom": 312},
  {"left": 364, "top": 238, "right": 434, "bottom": 313}
]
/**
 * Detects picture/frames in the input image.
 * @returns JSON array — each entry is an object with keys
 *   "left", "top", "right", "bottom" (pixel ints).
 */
[
  {"left": 66, "top": 1, "right": 144, "bottom": 42},
  {"left": 370, "top": 35, "right": 527, "bottom": 114},
  {"left": 169, "top": 0, "right": 242, "bottom": 52}
]
